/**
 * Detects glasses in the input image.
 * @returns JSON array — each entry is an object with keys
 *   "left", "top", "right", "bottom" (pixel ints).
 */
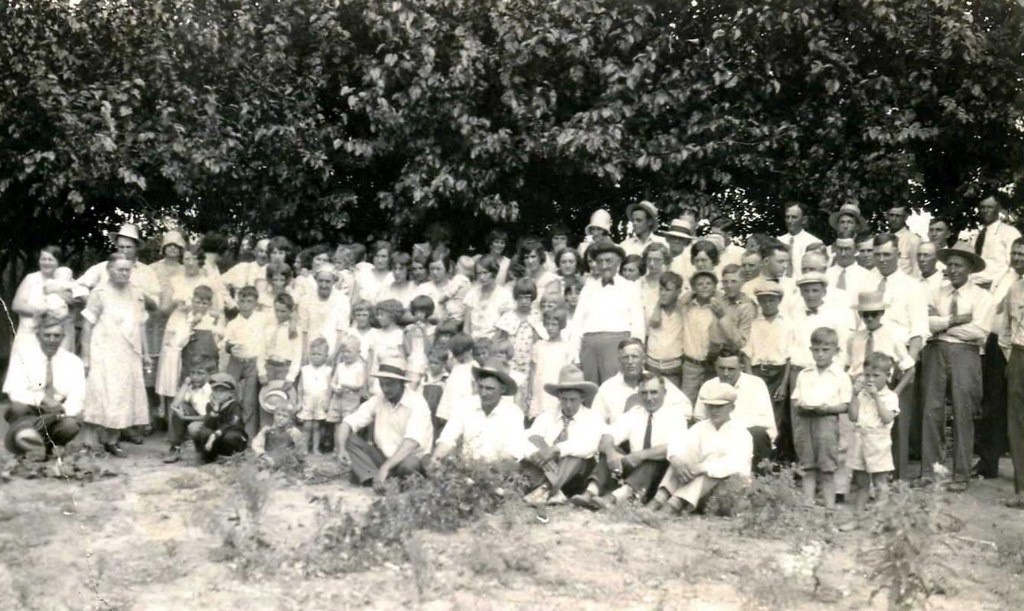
[{"left": 860, "top": 311, "right": 880, "bottom": 319}]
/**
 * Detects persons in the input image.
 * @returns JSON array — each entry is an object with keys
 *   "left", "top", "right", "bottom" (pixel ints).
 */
[
  {"left": 0, "top": 194, "right": 1024, "bottom": 521},
  {"left": 792, "top": 326, "right": 852, "bottom": 518}
]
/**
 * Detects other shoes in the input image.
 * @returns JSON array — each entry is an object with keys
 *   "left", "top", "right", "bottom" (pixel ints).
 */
[
  {"left": 14, "top": 422, "right": 323, "bottom": 472},
  {"left": 523, "top": 456, "right": 1023, "bottom": 528}
]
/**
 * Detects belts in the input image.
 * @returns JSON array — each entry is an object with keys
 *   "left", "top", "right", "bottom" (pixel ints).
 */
[
  {"left": 684, "top": 356, "right": 708, "bottom": 366},
  {"left": 754, "top": 364, "right": 780, "bottom": 371}
]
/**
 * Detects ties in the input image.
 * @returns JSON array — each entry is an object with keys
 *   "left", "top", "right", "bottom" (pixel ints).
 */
[
  {"left": 865, "top": 332, "right": 873, "bottom": 359},
  {"left": 45, "top": 355, "right": 54, "bottom": 397},
  {"left": 643, "top": 412, "right": 653, "bottom": 450},
  {"left": 877, "top": 277, "right": 887, "bottom": 295},
  {"left": 949, "top": 291, "right": 958, "bottom": 316},
  {"left": 975, "top": 227, "right": 987, "bottom": 255},
  {"left": 787, "top": 235, "right": 796, "bottom": 277}
]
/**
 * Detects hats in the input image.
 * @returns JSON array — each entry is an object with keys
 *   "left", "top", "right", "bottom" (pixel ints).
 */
[
  {"left": 661, "top": 219, "right": 696, "bottom": 240},
  {"left": 829, "top": 204, "right": 867, "bottom": 233},
  {"left": 936, "top": 241, "right": 986, "bottom": 273},
  {"left": 590, "top": 237, "right": 626, "bottom": 261},
  {"left": 851, "top": 291, "right": 891, "bottom": 313},
  {"left": 208, "top": 356, "right": 598, "bottom": 414},
  {"left": 626, "top": 200, "right": 659, "bottom": 232},
  {"left": 585, "top": 209, "right": 611, "bottom": 237},
  {"left": 754, "top": 281, "right": 784, "bottom": 298},
  {"left": 108, "top": 223, "right": 146, "bottom": 251},
  {"left": 699, "top": 382, "right": 737, "bottom": 405},
  {"left": 160, "top": 230, "right": 186, "bottom": 256},
  {"left": 796, "top": 271, "right": 828, "bottom": 287}
]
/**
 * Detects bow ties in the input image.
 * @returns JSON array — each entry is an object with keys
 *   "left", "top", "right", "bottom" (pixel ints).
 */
[{"left": 601, "top": 277, "right": 614, "bottom": 287}]
